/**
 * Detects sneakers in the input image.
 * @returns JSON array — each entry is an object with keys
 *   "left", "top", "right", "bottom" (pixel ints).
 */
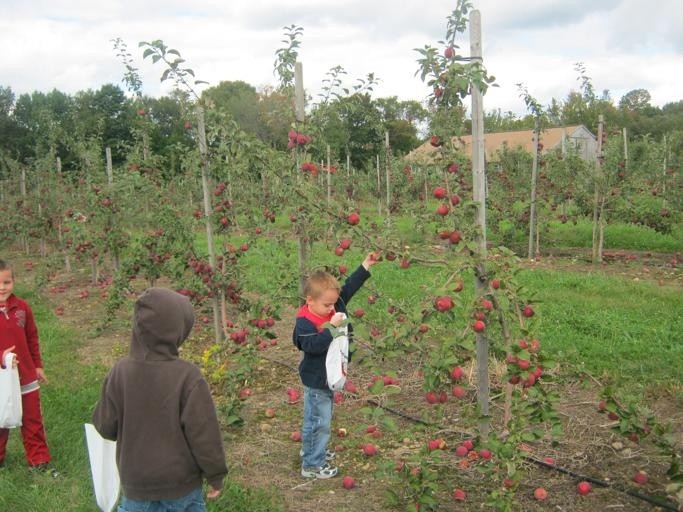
[
  {"left": 301, "top": 463, "right": 339, "bottom": 479},
  {"left": 299, "top": 446, "right": 338, "bottom": 460}
]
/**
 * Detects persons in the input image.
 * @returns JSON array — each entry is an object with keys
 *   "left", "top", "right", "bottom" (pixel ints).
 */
[
  {"left": 92, "top": 286, "right": 229, "bottom": 512},
  {"left": 0, "top": 259, "right": 54, "bottom": 473},
  {"left": 292, "top": 249, "right": 382, "bottom": 480}
]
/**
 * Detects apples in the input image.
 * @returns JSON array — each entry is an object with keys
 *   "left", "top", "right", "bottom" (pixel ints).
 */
[{"left": 1, "top": 47, "right": 680, "bottom": 511}]
